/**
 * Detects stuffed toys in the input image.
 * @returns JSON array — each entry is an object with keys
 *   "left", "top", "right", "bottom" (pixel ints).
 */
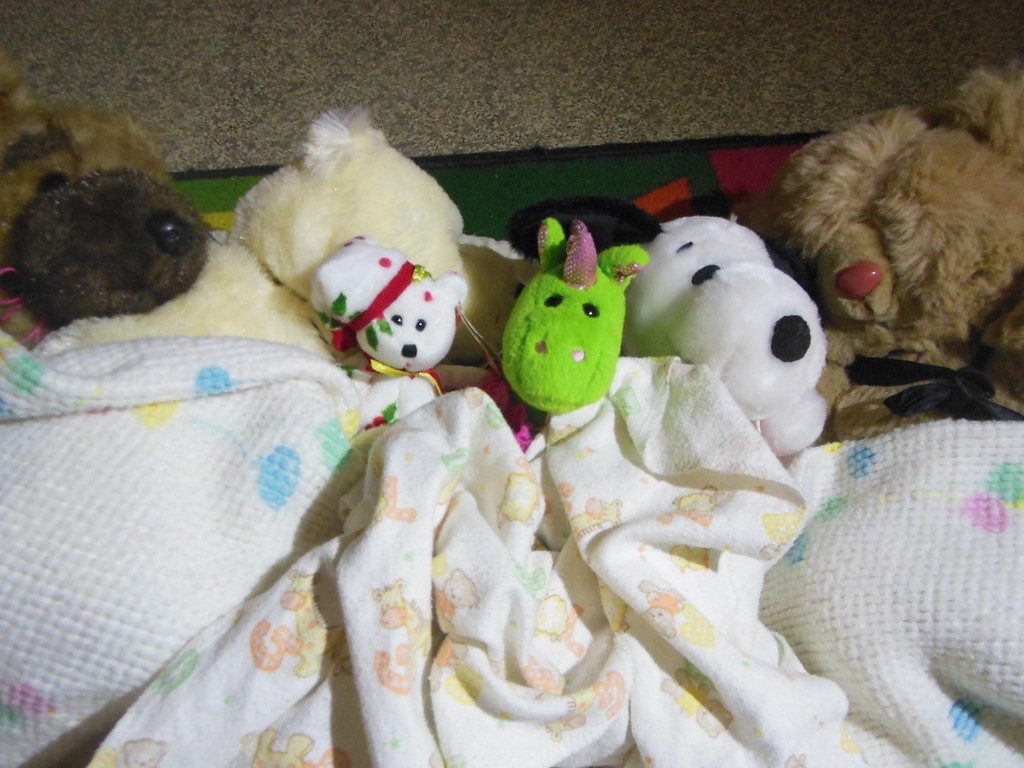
[
  {"left": 506, "top": 156, "right": 829, "bottom": 457},
  {"left": 0, "top": 46, "right": 652, "bottom": 454},
  {"left": 768, "top": 62, "right": 1024, "bottom": 440}
]
